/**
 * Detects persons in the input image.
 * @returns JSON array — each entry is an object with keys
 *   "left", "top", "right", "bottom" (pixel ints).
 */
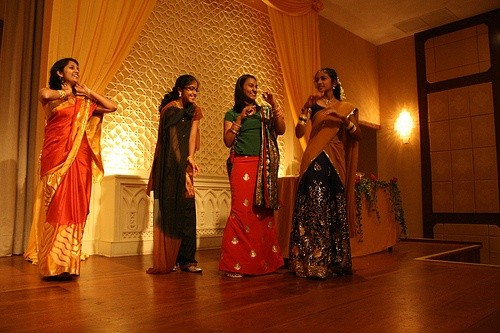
[
  {"left": 289, "top": 68, "right": 360, "bottom": 279},
  {"left": 39, "top": 58, "right": 118, "bottom": 277},
  {"left": 146, "top": 75, "right": 203, "bottom": 274},
  {"left": 219, "top": 74, "right": 286, "bottom": 278}
]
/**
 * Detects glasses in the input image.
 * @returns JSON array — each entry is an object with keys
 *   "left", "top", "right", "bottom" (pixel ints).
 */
[{"left": 185, "top": 85, "right": 199, "bottom": 92}]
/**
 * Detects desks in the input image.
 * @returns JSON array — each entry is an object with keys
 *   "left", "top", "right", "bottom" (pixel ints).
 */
[{"left": 276, "top": 175, "right": 399, "bottom": 258}]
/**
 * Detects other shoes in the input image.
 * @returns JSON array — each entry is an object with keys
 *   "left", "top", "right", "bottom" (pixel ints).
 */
[
  {"left": 172, "top": 264, "right": 203, "bottom": 274},
  {"left": 45, "top": 272, "right": 76, "bottom": 281},
  {"left": 224, "top": 270, "right": 243, "bottom": 278}
]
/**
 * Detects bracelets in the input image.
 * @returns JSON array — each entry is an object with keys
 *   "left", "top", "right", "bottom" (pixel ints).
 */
[
  {"left": 273, "top": 106, "right": 283, "bottom": 118},
  {"left": 87, "top": 88, "right": 90, "bottom": 96},
  {"left": 298, "top": 114, "right": 307, "bottom": 125},
  {"left": 231, "top": 121, "right": 241, "bottom": 134},
  {"left": 58, "top": 89, "right": 65, "bottom": 99},
  {"left": 346, "top": 121, "right": 357, "bottom": 134}
]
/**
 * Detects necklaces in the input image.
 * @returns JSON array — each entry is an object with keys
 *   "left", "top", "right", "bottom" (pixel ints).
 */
[{"left": 322, "top": 97, "right": 335, "bottom": 105}]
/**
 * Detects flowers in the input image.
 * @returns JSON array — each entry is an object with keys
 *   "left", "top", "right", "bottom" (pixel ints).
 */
[{"left": 354, "top": 173, "right": 408, "bottom": 241}]
